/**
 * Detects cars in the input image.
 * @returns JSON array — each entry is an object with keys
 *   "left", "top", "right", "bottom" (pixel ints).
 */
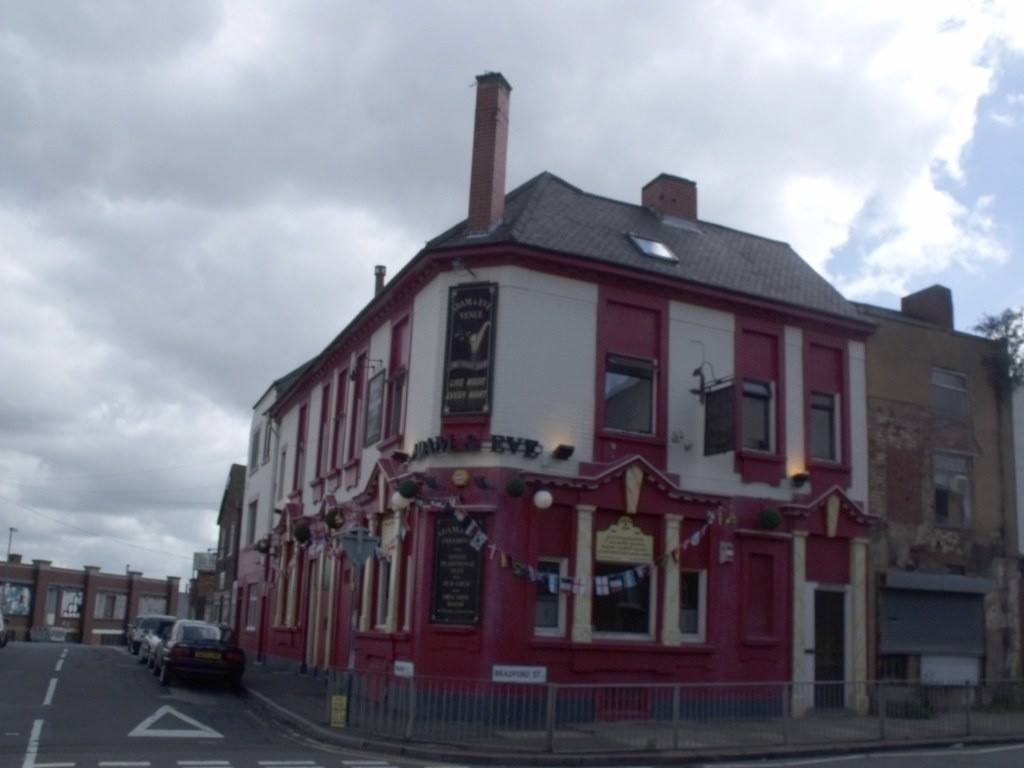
[
  {"left": 126, "top": 612, "right": 178, "bottom": 669},
  {"left": 0, "top": 611, "right": 12, "bottom": 647},
  {"left": 152, "top": 618, "right": 245, "bottom": 695}
]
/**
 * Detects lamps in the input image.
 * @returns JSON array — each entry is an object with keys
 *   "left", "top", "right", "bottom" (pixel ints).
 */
[
  {"left": 391, "top": 448, "right": 409, "bottom": 464},
  {"left": 793, "top": 471, "right": 810, "bottom": 487},
  {"left": 534, "top": 481, "right": 554, "bottom": 510},
  {"left": 323, "top": 504, "right": 348, "bottom": 528},
  {"left": 451, "top": 256, "right": 478, "bottom": 280},
  {"left": 393, "top": 480, "right": 409, "bottom": 509},
  {"left": 295, "top": 522, "right": 314, "bottom": 548},
  {"left": 256, "top": 539, "right": 272, "bottom": 554},
  {"left": 348, "top": 357, "right": 382, "bottom": 379},
  {"left": 554, "top": 445, "right": 574, "bottom": 460}
]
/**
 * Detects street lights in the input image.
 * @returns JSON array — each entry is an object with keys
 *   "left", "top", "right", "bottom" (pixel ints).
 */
[{"left": 6, "top": 526, "right": 20, "bottom": 563}]
